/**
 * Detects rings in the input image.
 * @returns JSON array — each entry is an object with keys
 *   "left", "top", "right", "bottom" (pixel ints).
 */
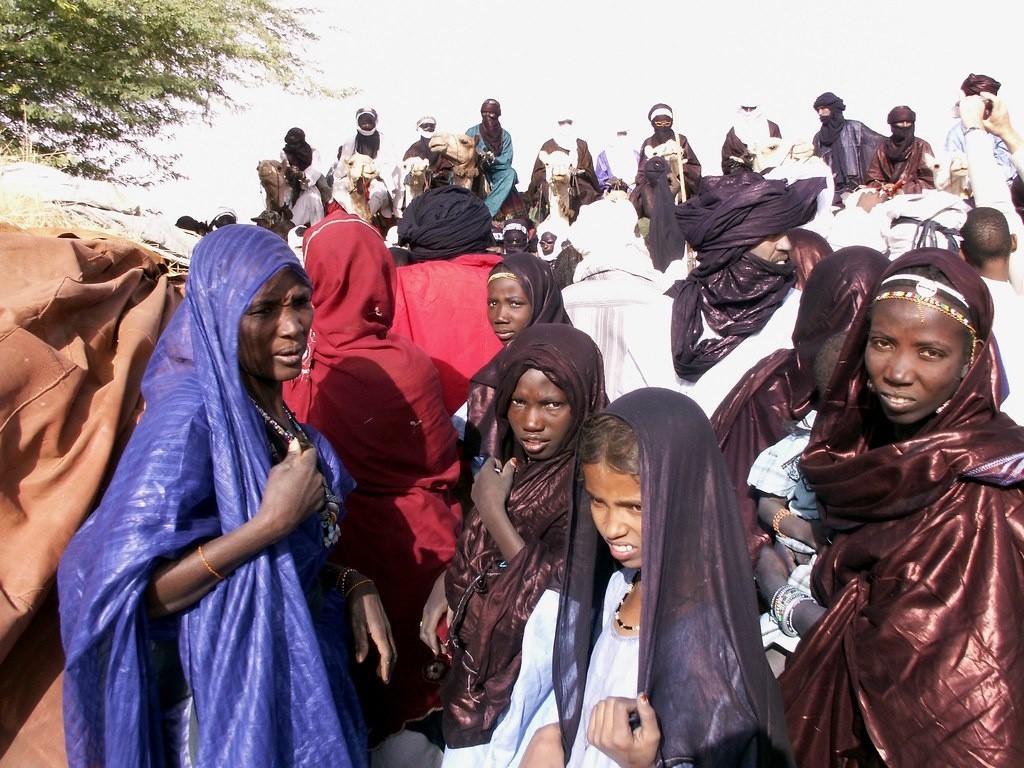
[{"left": 420, "top": 621, "right": 423, "bottom": 627}]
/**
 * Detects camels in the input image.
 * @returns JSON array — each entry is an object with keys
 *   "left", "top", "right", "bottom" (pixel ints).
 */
[
  {"left": 333, "top": 153, "right": 394, "bottom": 229},
  {"left": 925, "top": 150, "right": 966, "bottom": 190},
  {"left": 394, "top": 157, "right": 428, "bottom": 218},
  {"left": 644, "top": 139, "right": 687, "bottom": 207},
  {"left": 429, "top": 131, "right": 530, "bottom": 230},
  {"left": 535, "top": 149, "right": 575, "bottom": 244},
  {"left": 729, "top": 136, "right": 814, "bottom": 180},
  {"left": 257, "top": 159, "right": 323, "bottom": 229}
]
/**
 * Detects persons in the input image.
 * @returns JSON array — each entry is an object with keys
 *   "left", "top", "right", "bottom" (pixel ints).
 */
[{"left": 57, "top": 73, "right": 1024, "bottom": 768}]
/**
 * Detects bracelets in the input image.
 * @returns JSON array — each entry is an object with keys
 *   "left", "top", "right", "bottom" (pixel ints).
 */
[
  {"left": 773, "top": 509, "right": 796, "bottom": 538},
  {"left": 770, "top": 585, "right": 817, "bottom": 637},
  {"left": 199, "top": 546, "right": 226, "bottom": 580},
  {"left": 965, "top": 126, "right": 980, "bottom": 135},
  {"left": 346, "top": 579, "right": 373, "bottom": 597},
  {"left": 336, "top": 567, "right": 358, "bottom": 594}
]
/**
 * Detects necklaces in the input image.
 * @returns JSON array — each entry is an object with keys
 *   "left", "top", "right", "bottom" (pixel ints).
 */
[
  {"left": 247, "top": 396, "right": 341, "bottom": 548},
  {"left": 615, "top": 569, "right": 640, "bottom": 631}
]
